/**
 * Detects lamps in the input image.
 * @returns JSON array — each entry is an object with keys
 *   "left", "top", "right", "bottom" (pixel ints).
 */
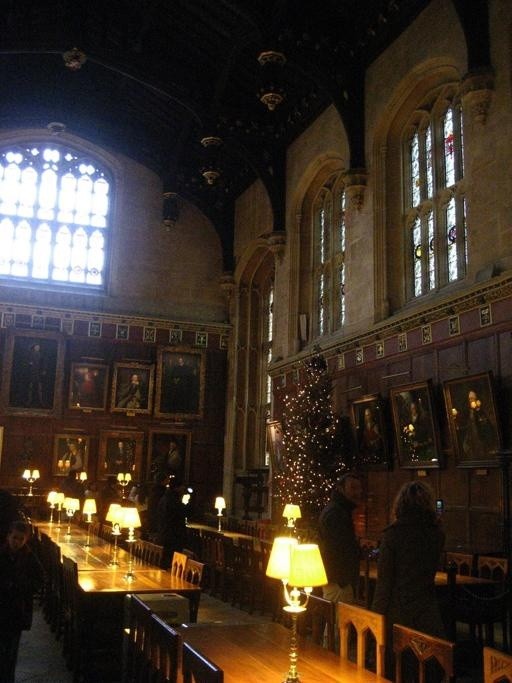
[
  {"left": 282, "top": 505, "right": 301, "bottom": 537},
  {"left": 46, "top": 491, "right": 169, "bottom": 586},
  {"left": 215, "top": 497, "right": 227, "bottom": 534},
  {"left": 182, "top": 489, "right": 195, "bottom": 526}
]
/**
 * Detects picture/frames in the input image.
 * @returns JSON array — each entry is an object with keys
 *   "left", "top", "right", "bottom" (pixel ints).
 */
[
  {"left": 344, "top": 389, "right": 398, "bottom": 476},
  {"left": 439, "top": 370, "right": 506, "bottom": 471},
  {"left": 0, "top": 318, "right": 208, "bottom": 493},
  {"left": 264, "top": 418, "right": 288, "bottom": 477},
  {"left": 386, "top": 377, "right": 444, "bottom": 473}
]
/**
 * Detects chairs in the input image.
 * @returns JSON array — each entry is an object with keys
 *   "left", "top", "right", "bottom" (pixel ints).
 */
[
  {"left": 124, "top": 593, "right": 394, "bottom": 682},
  {"left": 181, "top": 510, "right": 512, "bottom": 683},
  {"left": 1, "top": 507, "right": 205, "bottom": 671}
]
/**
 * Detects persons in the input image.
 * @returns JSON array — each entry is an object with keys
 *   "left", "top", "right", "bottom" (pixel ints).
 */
[
  {"left": 2, "top": 521, "right": 45, "bottom": 683},
  {"left": 362, "top": 406, "right": 381, "bottom": 454},
  {"left": 403, "top": 400, "right": 434, "bottom": 459},
  {"left": 21, "top": 341, "right": 45, "bottom": 408},
  {"left": 78, "top": 370, "right": 99, "bottom": 405},
  {"left": 462, "top": 389, "right": 497, "bottom": 456},
  {"left": 60, "top": 441, "right": 82, "bottom": 470},
  {"left": 153, "top": 439, "right": 182, "bottom": 469},
  {"left": 315, "top": 472, "right": 368, "bottom": 650},
  {"left": 112, "top": 440, "right": 127, "bottom": 470},
  {"left": 370, "top": 479, "right": 447, "bottom": 680},
  {"left": 118, "top": 374, "right": 142, "bottom": 407},
  {"left": 172, "top": 356, "right": 193, "bottom": 412},
  {"left": 62, "top": 470, "right": 194, "bottom": 569}
]
